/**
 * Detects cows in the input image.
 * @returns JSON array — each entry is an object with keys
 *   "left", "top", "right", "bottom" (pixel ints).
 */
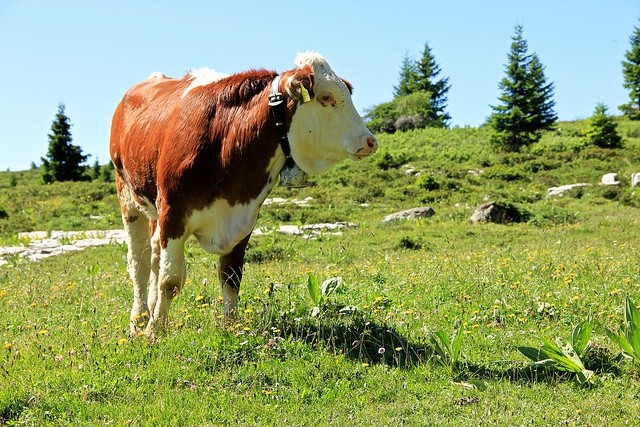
[{"left": 109, "top": 50, "right": 379, "bottom": 345}]
